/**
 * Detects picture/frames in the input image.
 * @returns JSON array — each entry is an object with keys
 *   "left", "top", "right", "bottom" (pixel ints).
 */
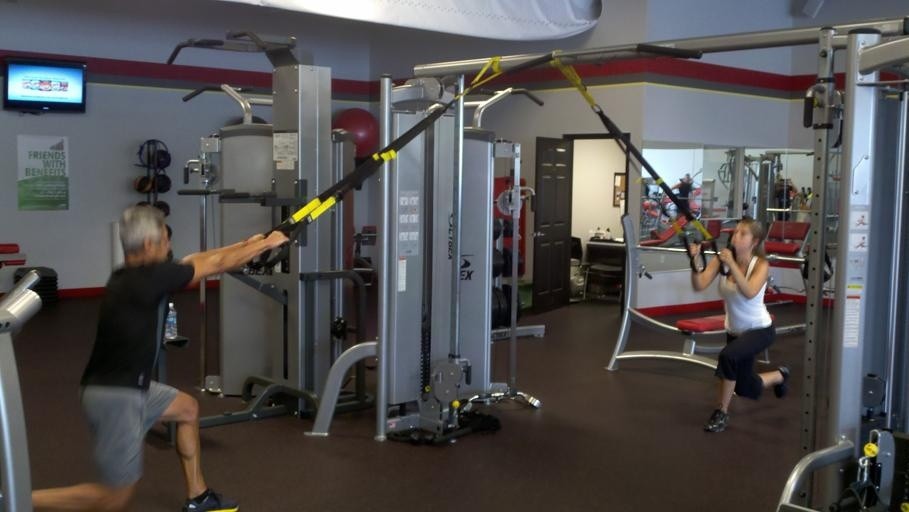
[{"left": 614, "top": 173, "right": 626, "bottom": 207}]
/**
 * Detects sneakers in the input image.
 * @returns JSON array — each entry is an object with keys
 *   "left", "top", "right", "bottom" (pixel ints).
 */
[
  {"left": 774, "top": 366, "right": 790, "bottom": 398},
  {"left": 182, "top": 488, "right": 240, "bottom": 512},
  {"left": 703, "top": 409, "right": 730, "bottom": 433}
]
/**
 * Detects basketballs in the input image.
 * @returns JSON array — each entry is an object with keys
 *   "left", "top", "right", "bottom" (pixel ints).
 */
[{"left": 135, "top": 175, "right": 152, "bottom": 192}]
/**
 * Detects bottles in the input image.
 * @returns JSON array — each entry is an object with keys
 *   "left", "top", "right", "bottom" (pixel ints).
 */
[{"left": 164, "top": 303, "right": 177, "bottom": 339}]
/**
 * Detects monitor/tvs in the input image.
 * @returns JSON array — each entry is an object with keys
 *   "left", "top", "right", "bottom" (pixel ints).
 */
[{"left": 3, "top": 61, "right": 88, "bottom": 115}]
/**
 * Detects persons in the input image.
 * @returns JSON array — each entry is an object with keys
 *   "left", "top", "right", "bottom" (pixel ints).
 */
[
  {"left": 800, "top": 186, "right": 807, "bottom": 200},
  {"left": 807, "top": 186, "right": 813, "bottom": 202},
  {"left": 668, "top": 167, "right": 703, "bottom": 199},
  {"left": 29, "top": 205, "right": 293, "bottom": 511},
  {"left": 685, "top": 218, "right": 790, "bottom": 433},
  {"left": 776, "top": 179, "right": 797, "bottom": 222}
]
